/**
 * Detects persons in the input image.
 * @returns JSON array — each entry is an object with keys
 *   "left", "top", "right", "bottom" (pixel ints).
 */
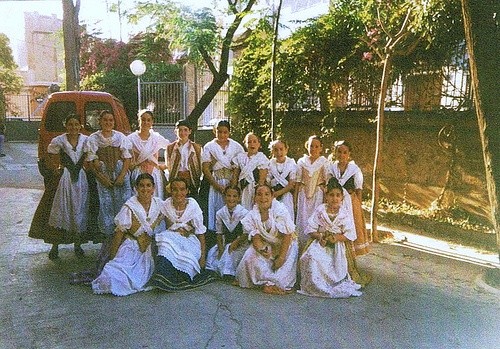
[
  {"left": 296, "top": 180, "right": 371, "bottom": 298},
  {"left": 70, "top": 173, "right": 162, "bottom": 296},
  {"left": 204, "top": 185, "right": 249, "bottom": 280},
  {"left": 0, "top": 120, "right": 6, "bottom": 157},
  {"left": 236, "top": 181, "right": 299, "bottom": 295},
  {"left": 27, "top": 111, "right": 371, "bottom": 261},
  {"left": 147, "top": 177, "right": 215, "bottom": 293}
]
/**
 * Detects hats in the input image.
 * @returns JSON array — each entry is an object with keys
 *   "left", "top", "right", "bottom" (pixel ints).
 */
[{"left": 176, "top": 120, "right": 189, "bottom": 128}]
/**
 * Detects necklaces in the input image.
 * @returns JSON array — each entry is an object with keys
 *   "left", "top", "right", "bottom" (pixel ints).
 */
[{"left": 331, "top": 210, "right": 337, "bottom": 213}]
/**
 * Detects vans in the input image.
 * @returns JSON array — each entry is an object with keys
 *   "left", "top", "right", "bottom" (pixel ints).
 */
[{"left": 37, "top": 91, "right": 131, "bottom": 188}]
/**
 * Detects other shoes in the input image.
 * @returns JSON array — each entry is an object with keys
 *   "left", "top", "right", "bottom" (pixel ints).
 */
[
  {"left": 74, "top": 245, "right": 85, "bottom": 258},
  {"left": 49, "top": 246, "right": 59, "bottom": 260}
]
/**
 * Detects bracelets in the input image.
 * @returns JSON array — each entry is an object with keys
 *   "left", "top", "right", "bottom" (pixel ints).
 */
[{"left": 235, "top": 239, "right": 240, "bottom": 243}]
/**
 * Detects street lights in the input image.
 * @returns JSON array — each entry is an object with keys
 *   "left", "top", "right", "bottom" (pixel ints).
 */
[{"left": 131, "top": 60, "right": 146, "bottom": 112}]
